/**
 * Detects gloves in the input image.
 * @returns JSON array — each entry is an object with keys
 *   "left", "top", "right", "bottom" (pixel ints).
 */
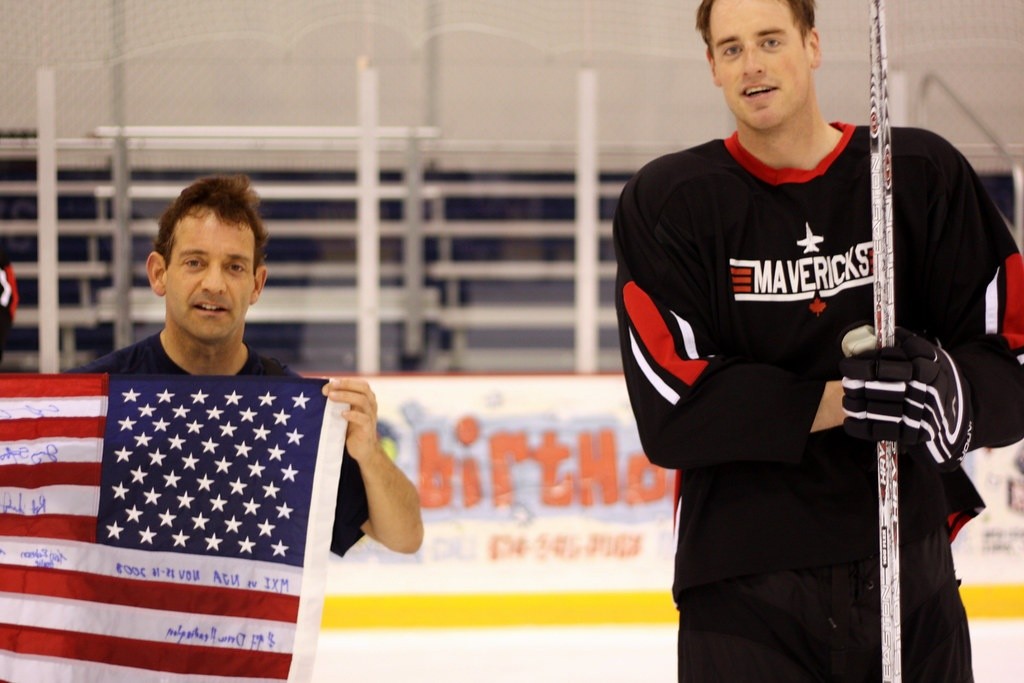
[{"left": 839, "top": 319, "right": 974, "bottom": 473}]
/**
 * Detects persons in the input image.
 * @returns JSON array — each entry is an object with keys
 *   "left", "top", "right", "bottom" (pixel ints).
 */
[
  {"left": 62, "top": 176, "right": 423, "bottom": 557},
  {"left": 609, "top": 0, "right": 1024, "bottom": 683}
]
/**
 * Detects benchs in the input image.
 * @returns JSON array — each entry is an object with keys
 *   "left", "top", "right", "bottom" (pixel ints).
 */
[{"left": 0, "top": 127, "right": 645, "bottom": 372}]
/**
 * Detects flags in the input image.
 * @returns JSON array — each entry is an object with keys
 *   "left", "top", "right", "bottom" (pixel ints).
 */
[{"left": 0, "top": 373, "right": 353, "bottom": 683}]
